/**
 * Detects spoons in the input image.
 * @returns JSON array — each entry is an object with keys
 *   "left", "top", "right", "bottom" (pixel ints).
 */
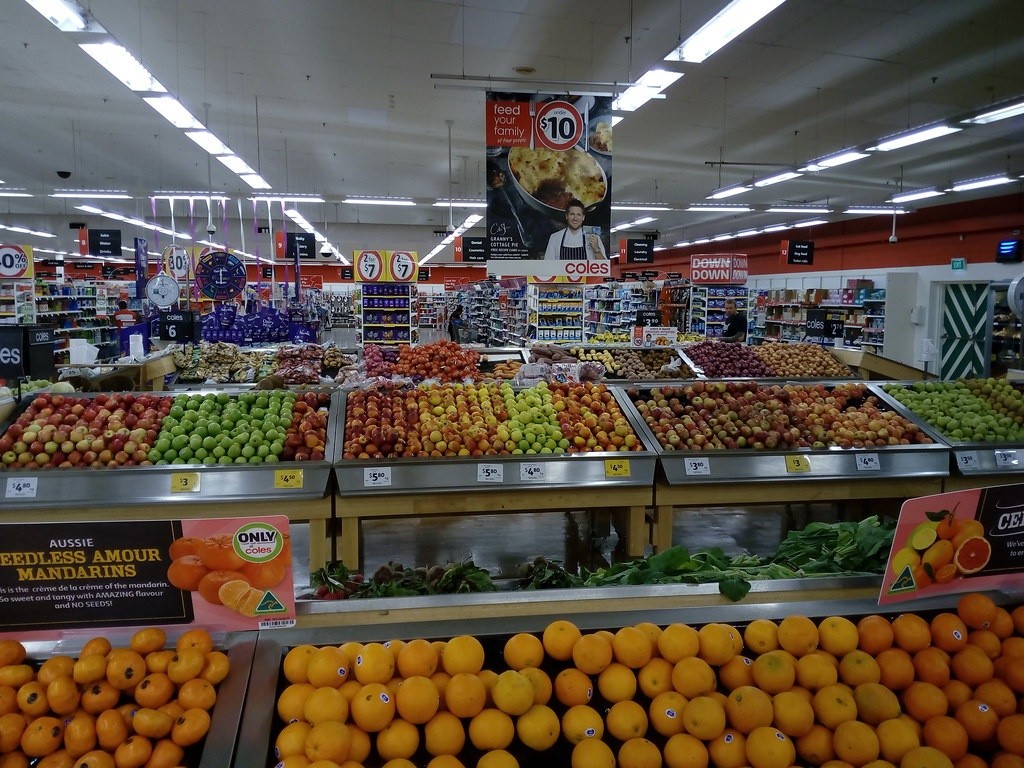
[{"left": 486, "top": 162, "right": 536, "bottom": 248}]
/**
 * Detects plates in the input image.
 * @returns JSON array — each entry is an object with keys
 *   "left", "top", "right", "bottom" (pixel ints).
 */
[
  {"left": 579, "top": 115, "right": 611, "bottom": 155},
  {"left": 507, "top": 139, "right": 607, "bottom": 217}
]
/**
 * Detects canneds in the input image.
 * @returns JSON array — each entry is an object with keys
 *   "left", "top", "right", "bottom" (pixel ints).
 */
[{"left": 363, "top": 284, "right": 409, "bottom": 308}]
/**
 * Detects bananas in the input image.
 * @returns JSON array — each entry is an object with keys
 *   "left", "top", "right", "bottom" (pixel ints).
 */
[
  {"left": 589, "top": 330, "right": 629, "bottom": 343},
  {"left": 676, "top": 331, "right": 710, "bottom": 342}
]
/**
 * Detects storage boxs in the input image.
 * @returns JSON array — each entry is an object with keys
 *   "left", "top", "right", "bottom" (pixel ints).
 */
[
  {"left": 0, "top": 346, "right": 1024, "bottom": 508},
  {"left": 767, "top": 278, "right": 875, "bottom": 342}
]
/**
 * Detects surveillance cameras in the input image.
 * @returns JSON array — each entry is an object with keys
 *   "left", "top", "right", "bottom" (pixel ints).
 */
[
  {"left": 446, "top": 225, "right": 456, "bottom": 234},
  {"left": 318, "top": 245, "right": 333, "bottom": 257},
  {"left": 56, "top": 171, "right": 71, "bottom": 178},
  {"left": 206, "top": 224, "right": 216, "bottom": 235},
  {"left": 889, "top": 236, "right": 897, "bottom": 244}
]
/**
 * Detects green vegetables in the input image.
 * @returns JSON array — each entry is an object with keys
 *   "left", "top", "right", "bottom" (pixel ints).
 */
[{"left": 579, "top": 513, "right": 895, "bottom": 604}]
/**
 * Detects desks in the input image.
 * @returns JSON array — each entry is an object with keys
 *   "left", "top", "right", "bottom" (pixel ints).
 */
[
  {"left": 0, "top": 493, "right": 331, "bottom": 590},
  {"left": 336, "top": 484, "right": 654, "bottom": 575},
  {"left": 525, "top": 283, "right": 586, "bottom": 343},
  {"left": 656, "top": 474, "right": 944, "bottom": 572}
]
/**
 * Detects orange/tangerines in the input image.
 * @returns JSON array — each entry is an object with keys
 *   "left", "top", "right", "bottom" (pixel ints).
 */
[
  {"left": 273, "top": 594, "right": 1024, "bottom": 768},
  {"left": 891, "top": 515, "right": 991, "bottom": 588},
  {"left": 0, "top": 627, "right": 229, "bottom": 768},
  {"left": 167, "top": 533, "right": 292, "bottom": 617}
]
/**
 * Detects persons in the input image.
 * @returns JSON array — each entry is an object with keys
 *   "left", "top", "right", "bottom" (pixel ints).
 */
[
  {"left": 447, "top": 305, "right": 469, "bottom": 344},
  {"left": 111, "top": 301, "right": 139, "bottom": 354},
  {"left": 715, "top": 298, "right": 747, "bottom": 342},
  {"left": 544, "top": 198, "right": 607, "bottom": 260}
]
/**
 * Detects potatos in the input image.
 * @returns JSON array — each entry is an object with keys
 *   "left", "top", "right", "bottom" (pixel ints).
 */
[
  {"left": 529, "top": 342, "right": 694, "bottom": 379},
  {"left": 363, "top": 337, "right": 525, "bottom": 382},
  {"left": 683, "top": 341, "right": 853, "bottom": 379}
]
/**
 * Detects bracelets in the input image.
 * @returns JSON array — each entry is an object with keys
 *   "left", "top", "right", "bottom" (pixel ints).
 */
[{"left": 595, "top": 247, "right": 601, "bottom": 254}]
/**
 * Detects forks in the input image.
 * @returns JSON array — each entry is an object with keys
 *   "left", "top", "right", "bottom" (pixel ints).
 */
[{"left": 528, "top": 99, "right": 537, "bottom": 150}]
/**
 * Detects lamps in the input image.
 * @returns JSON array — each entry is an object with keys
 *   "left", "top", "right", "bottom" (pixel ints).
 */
[
  {"left": 0, "top": 0, "right": 490, "bottom": 271},
  {"left": 610, "top": 0, "right": 1024, "bottom": 260}
]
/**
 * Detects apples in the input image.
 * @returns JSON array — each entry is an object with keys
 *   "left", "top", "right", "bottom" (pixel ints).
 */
[
  {"left": 627, "top": 377, "right": 1024, "bottom": 450},
  {"left": 343, "top": 380, "right": 645, "bottom": 459},
  {"left": 0, "top": 391, "right": 330, "bottom": 472}
]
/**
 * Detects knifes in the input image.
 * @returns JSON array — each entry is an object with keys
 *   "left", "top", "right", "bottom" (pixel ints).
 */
[{"left": 584, "top": 102, "right": 589, "bottom": 152}]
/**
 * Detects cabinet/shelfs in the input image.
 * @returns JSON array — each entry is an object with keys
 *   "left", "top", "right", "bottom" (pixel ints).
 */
[
  {"left": 360, "top": 284, "right": 411, "bottom": 350},
  {"left": 29, "top": 291, "right": 122, "bottom": 374},
  {"left": 584, "top": 277, "right": 698, "bottom": 339},
  {"left": 353, "top": 300, "right": 362, "bottom": 350},
  {"left": 0, "top": 296, "right": 20, "bottom": 324},
  {"left": 444, "top": 296, "right": 528, "bottom": 347},
  {"left": 410, "top": 294, "right": 447, "bottom": 328},
  {"left": 749, "top": 287, "right": 885, "bottom": 359},
  {"left": 690, "top": 284, "right": 751, "bottom": 343}
]
